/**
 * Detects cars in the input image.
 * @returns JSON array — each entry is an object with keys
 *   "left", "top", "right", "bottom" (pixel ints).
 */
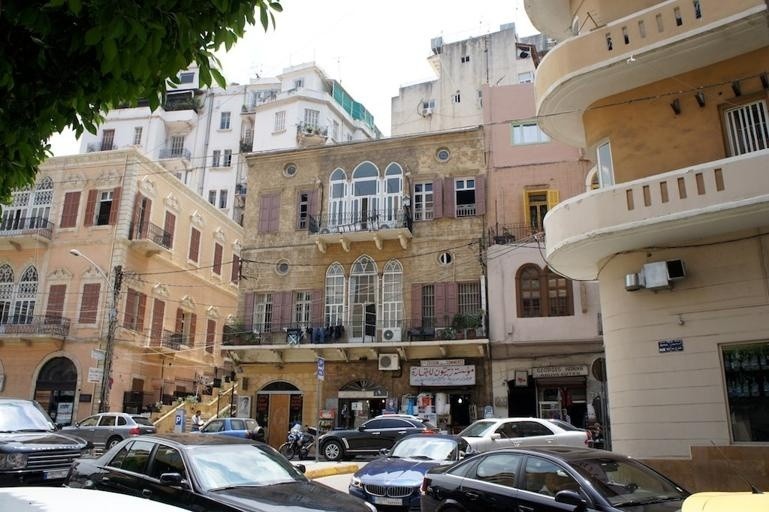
[
  {"left": 678, "top": 437, "right": 769, "bottom": 512},
  {"left": 410, "top": 443, "right": 693, "bottom": 512}
]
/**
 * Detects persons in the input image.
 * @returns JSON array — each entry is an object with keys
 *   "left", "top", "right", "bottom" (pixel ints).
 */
[
  {"left": 538, "top": 470, "right": 561, "bottom": 497},
  {"left": 191, "top": 410, "right": 204, "bottom": 431},
  {"left": 239, "top": 397, "right": 249, "bottom": 415}
]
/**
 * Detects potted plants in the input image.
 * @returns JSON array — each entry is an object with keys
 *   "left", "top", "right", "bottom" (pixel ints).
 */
[
  {"left": 462, "top": 309, "right": 484, "bottom": 337},
  {"left": 451, "top": 312, "right": 464, "bottom": 339}
]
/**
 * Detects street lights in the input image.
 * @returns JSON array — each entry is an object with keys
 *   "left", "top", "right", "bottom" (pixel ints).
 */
[{"left": 67, "top": 247, "right": 122, "bottom": 409}]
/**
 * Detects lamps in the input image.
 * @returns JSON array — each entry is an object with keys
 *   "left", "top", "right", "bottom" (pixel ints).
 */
[{"left": 670, "top": 74, "right": 769, "bottom": 115}]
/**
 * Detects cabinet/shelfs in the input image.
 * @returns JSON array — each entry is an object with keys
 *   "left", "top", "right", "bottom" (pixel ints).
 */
[{"left": 539, "top": 401, "right": 562, "bottom": 421}]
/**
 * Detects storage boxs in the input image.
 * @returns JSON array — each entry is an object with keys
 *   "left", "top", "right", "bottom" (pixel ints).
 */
[{"left": 319, "top": 408, "right": 336, "bottom": 419}]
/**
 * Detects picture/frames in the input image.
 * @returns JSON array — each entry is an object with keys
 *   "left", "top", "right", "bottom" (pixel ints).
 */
[
  {"left": 236, "top": 396, "right": 252, "bottom": 418},
  {"left": 515, "top": 371, "right": 528, "bottom": 387}
]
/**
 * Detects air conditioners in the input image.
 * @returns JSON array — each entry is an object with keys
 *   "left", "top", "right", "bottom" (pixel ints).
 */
[
  {"left": 421, "top": 108, "right": 432, "bottom": 116},
  {"left": 435, "top": 328, "right": 451, "bottom": 340},
  {"left": 381, "top": 327, "right": 401, "bottom": 342},
  {"left": 378, "top": 354, "right": 400, "bottom": 370}
]
